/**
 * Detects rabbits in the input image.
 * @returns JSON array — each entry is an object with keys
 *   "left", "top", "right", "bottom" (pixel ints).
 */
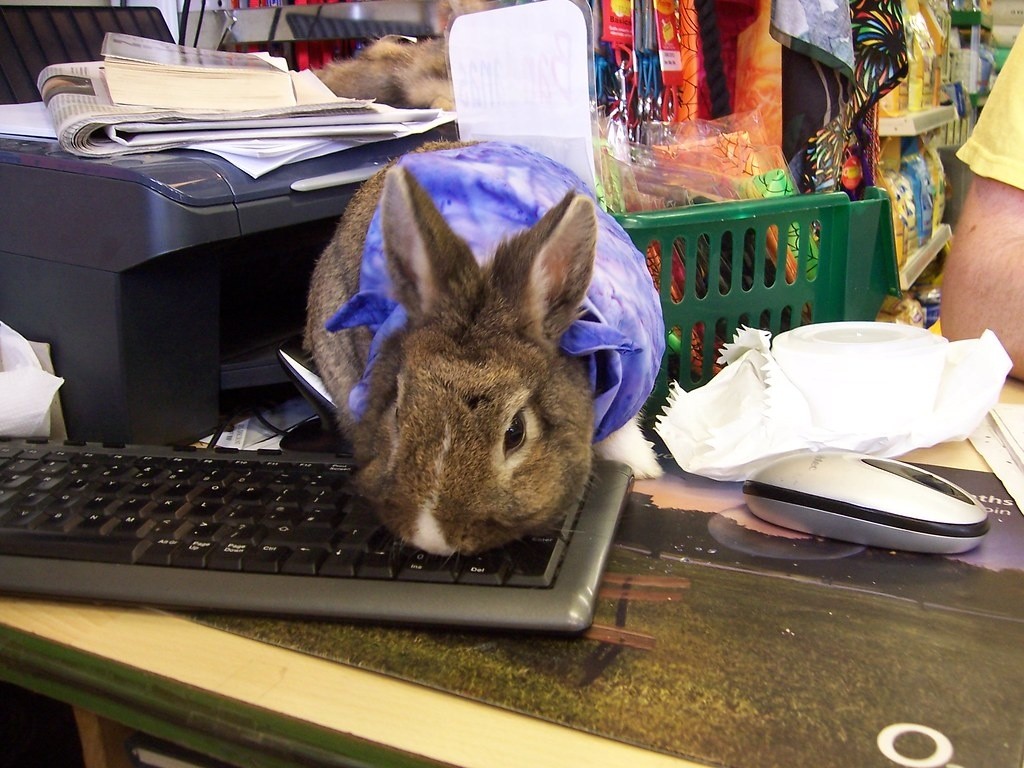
[{"left": 305, "top": 140, "right": 667, "bottom": 559}]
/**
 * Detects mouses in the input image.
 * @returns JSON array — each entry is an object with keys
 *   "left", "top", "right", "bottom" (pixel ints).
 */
[{"left": 740, "top": 450, "right": 993, "bottom": 554}]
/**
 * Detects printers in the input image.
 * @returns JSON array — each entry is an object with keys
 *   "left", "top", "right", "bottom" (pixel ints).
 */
[{"left": 1, "top": 116, "right": 461, "bottom": 440}]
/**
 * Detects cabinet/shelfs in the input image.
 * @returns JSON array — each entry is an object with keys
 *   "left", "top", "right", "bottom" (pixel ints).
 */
[{"left": 878, "top": 0, "right": 996, "bottom": 334}]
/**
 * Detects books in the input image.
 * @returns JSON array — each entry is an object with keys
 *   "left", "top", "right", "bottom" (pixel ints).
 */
[{"left": 102, "top": 33, "right": 297, "bottom": 109}]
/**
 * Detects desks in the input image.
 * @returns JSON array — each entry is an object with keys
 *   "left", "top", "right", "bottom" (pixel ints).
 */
[{"left": 0, "top": 374, "right": 1024, "bottom": 768}]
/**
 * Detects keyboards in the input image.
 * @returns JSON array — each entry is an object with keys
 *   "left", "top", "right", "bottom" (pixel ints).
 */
[{"left": 0, "top": 434, "right": 634, "bottom": 633}]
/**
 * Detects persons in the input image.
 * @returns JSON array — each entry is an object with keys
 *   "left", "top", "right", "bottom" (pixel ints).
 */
[{"left": 938, "top": 22, "right": 1024, "bottom": 383}]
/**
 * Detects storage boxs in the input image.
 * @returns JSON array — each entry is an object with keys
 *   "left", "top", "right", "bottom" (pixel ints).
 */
[{"left": 606, "top": 183, "right": 903, "bottom": 436}]
